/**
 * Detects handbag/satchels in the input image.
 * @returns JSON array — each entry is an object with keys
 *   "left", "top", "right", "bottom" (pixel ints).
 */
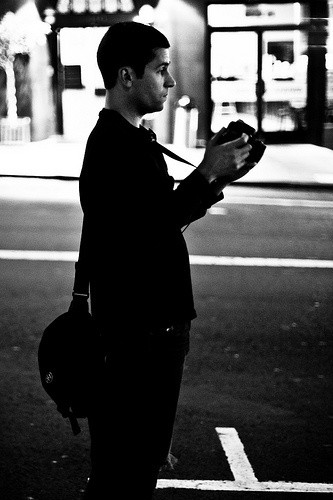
[{"left": 38, "top": 312, "right": 99, "bottom": 421}]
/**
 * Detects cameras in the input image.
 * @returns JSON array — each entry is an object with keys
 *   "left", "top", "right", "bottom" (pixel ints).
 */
[{"left": 221, "top": 119, "right": 265, "bottom": 165}]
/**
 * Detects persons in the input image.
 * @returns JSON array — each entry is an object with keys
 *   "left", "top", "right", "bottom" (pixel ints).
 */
[{"left": 74, "top": 22, "right": 262, "bottom": 500}]
